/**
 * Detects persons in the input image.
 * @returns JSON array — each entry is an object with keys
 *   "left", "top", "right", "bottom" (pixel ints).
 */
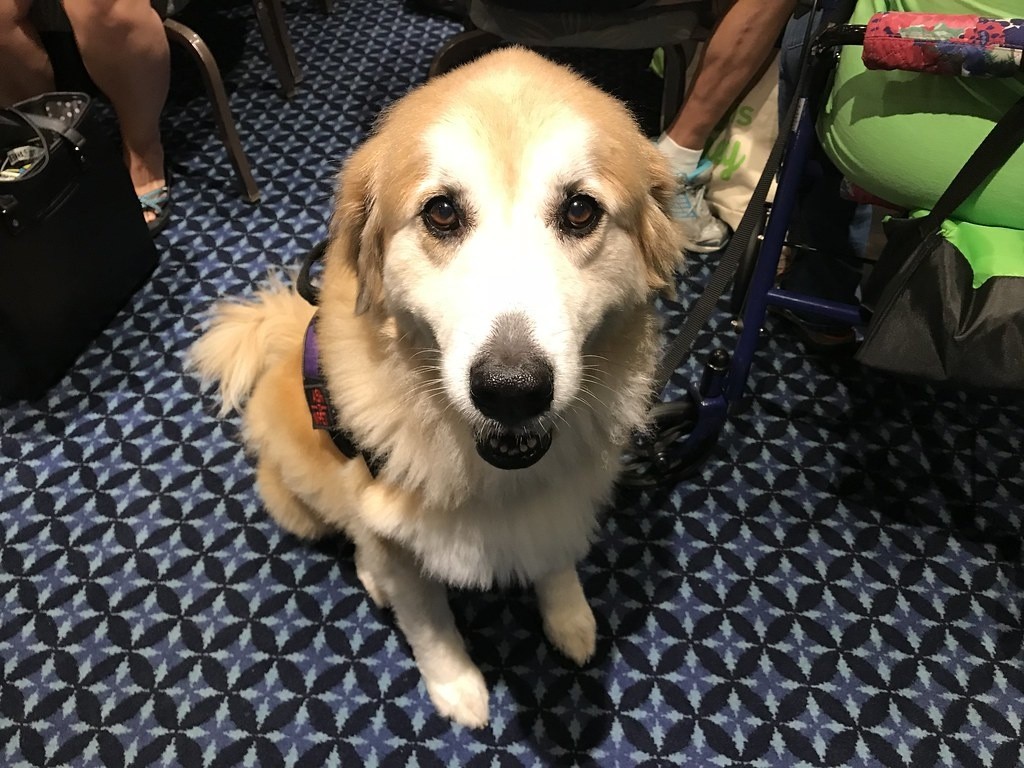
[
  {"left": 403, "top": 0, "right": 796, "bottom": 253},
  {"left": 781, "top": 0, "right": 1024, "bottom": 344},
  {"left": 0, "top": 0, "right": 172, "bottom": 238}
]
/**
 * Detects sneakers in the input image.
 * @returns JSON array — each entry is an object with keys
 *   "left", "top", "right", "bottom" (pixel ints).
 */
[{"left": 651, "top": 161, "right": 731, "bottom": 253}]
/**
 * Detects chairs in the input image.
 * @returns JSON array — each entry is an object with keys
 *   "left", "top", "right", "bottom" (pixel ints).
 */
[{"left": 618, "top": 0, "right": 1024, "bottom": 488}]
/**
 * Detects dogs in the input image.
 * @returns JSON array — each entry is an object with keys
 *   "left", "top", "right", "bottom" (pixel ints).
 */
[{"left": 182, "top": 43, "right": 688, "bottom": 729}]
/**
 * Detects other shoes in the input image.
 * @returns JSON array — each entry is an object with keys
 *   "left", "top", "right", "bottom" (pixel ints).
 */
[{"left": 772, "top": 306, "right": 856, "bottom": 347}]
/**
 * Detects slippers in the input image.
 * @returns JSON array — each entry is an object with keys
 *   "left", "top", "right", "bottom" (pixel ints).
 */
[{"left": 129, "top": 161, "right": 173, "bottom": 234}]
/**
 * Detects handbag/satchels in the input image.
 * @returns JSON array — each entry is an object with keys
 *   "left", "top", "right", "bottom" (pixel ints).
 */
[
  {"left": 852, "top": 215, "right": 1023, "bottom": 389},
  {"left": 0, "top": 92, "right": 160, "bottom": 408}
]
